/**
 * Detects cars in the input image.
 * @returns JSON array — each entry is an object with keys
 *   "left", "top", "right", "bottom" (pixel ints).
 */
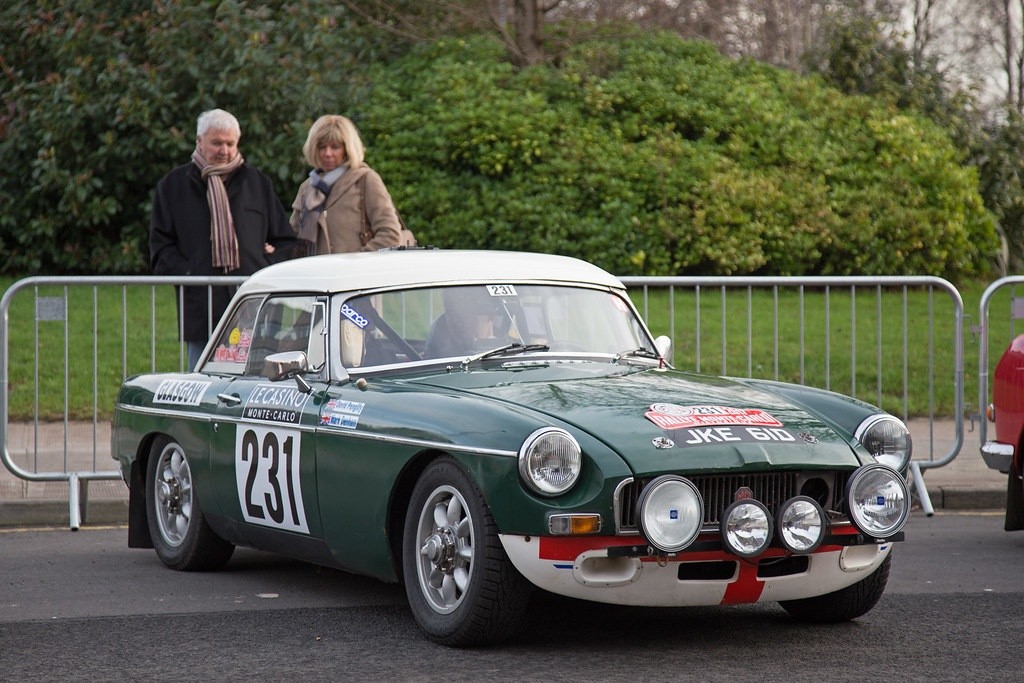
[{"left": 109, "top": 246, "right": 913, "bottom": 645}]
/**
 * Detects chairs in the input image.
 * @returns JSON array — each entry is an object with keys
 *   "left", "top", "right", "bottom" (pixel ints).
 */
[{"left": 422, "top": 314, "right": 456, "bottom": 358}]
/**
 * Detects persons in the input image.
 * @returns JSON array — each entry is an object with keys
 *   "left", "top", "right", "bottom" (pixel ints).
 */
[
  {"left": 289, "top": 113, "right": 402, "bottom": 254},
  {"left": 438, "top": 287, "right": 498, "bottom": 358},
  {"left": 148, "top": 109, "right": 296, "bottom": 373}
]
